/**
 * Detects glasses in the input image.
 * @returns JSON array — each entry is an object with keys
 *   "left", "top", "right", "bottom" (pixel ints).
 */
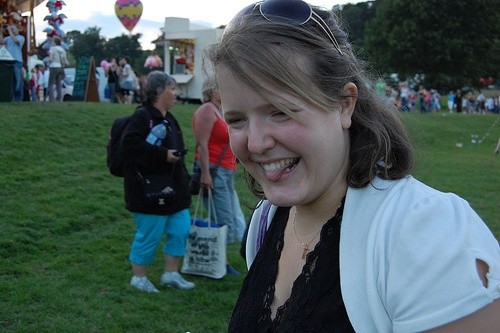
[{"left": 225, "top": 0, "right": 343, "bottom": 56}]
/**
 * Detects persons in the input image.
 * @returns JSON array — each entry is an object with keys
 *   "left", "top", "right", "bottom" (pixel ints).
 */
[
  {"left": 123, "top": 69, "right": 198, "bottom": 294},
  {"left": 47, "top": 37, "right": 67, "bottom": 102},
  {"left": 22, "top": 62, "right": 50, "bottom": 103},
  {"left": 370, "top": 69, "right": 500, "bottom": 117},
  {"left": 200, "top": 0, "right": 500, "bottom": 333},
  {"left": 0, "top": 24, "right": 26, "bottom": 102},
  {"left": 191, "top": 74, "right": 247, "bottom": 245},
  {"left": 99, "top": 49, "right": 164, "bottom": 105}
]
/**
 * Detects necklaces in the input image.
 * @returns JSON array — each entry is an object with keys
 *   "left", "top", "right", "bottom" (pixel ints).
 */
[{"left": 292, "top": 204, "right": 339, "bottom": 261}]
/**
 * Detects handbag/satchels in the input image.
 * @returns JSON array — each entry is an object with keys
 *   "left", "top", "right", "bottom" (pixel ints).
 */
[
  {"left": 191, "top": 161, "right": 218, "bottom": 195},
  {"left": 185, "top": 186, "right": 227, "bottom": 279},
  {"left": 60, "top": 67, "right": 64, "bottom": 80}
]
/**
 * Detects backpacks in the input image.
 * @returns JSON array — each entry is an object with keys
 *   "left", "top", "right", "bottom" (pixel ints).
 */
[{"left": 107, "top": 108, "right": 154, "bottom": 178}]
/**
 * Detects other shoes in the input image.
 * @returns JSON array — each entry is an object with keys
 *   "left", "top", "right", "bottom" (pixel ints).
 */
[
  {"left": 161, "top": 271, "right": 195, "bottom": 290},
  {"left": 130, "top": 276, "right": 159, "bottom": 294},
  {"left": 226, "top": 264, "right": 241, "bottom": 276}
]
